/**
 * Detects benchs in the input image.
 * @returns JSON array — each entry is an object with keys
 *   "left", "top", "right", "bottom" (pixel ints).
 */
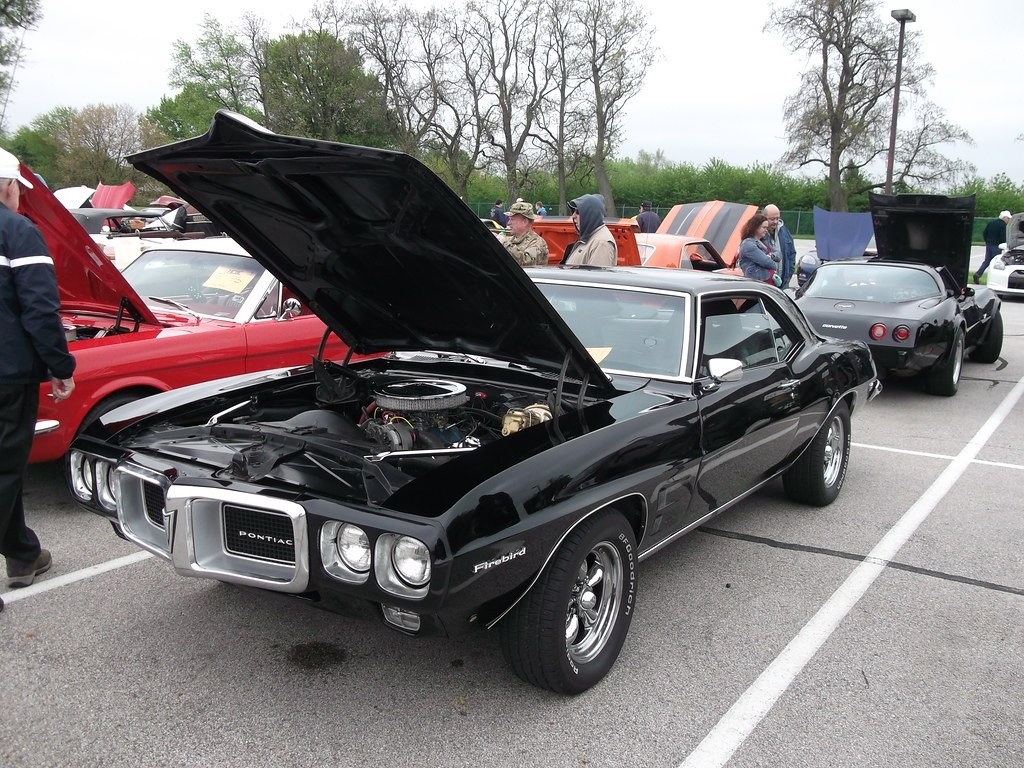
[{"left": 574, "top": 315, "right": 767, "bottom": 363}]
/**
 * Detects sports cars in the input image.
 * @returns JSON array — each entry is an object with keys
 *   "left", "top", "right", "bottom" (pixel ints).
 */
[
  {"left": 105, "top": 107, "right": 884, "bottom": 696},
  {"left": 987, "top": 211, "right": 1024, "bottom": 298},
  {"left": 772, "top": 189, "right": 1002, "bottom": 398},
  {"left": 14, "top": 181, "right": 761, "bottom": 482}
]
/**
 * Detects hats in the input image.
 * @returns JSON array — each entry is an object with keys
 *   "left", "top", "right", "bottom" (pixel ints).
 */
[
  {"left": 515, "top": 197, "right": 524, "bottom": 203},
  {"left": 0, "top": 148, "right": 34, "bottom": 189},
  {"left": 641, "top": 201, "right": 652, "bottom": 207},
  {"left": 566, "top": 201, "right": 577, "bottom": 211},
  {"left": 503, "top": 202, "right": 533, "bottom": 219},
  {"left": 998, "top": 210, "right": 1015, "bottom": 219}
]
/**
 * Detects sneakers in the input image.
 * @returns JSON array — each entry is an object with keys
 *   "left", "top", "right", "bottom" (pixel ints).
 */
[{"left": 6, "top": 550, "right": 51, "bottom": 585}]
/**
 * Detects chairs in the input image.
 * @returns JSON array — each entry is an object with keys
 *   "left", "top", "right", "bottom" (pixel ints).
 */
[{"left": 668, "top": 298, "right": 743, "bottom": 370}]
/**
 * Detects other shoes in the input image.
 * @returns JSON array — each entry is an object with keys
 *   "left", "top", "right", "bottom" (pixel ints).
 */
[{"left": 974, "top": 274, "right": 981, "bottom": 284}]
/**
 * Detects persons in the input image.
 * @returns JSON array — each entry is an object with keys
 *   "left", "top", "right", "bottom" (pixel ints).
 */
[
  {"left": 559, "top": 194, "right": 618, "bottom": 265},
  {"left": 536, "top": 201, "right": 547, "bottom": 216},
  {"left": 490, "top": 200, "right": 506, "bottom": 227},
  {"left": 506, "top": 198, "right": 525, "bottom": 221},
  {"left": 493, "top": 202, "right": 548, "bottom": 265},
  {"left": 632, "top": 201, "right": 660, "bottom": 233},
  {"left": 762, "top": 204, "right": 796, "bottom": 289},
  {"left": 0, "top": 148, "right": 76, "bottom": 611},
  {"left": 739, "top": 215, "right": 780, "bottom": 286},
  {"left": 973, "top": 211, "right": 1012, "bottom": 284}
]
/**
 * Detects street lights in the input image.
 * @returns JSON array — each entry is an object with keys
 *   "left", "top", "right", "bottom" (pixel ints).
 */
[{"left": 882, "top": 8, "right": 916, "bottom": 194}]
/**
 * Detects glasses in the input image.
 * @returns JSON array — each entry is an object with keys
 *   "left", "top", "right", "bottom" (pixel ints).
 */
[{"left": 571, "top": 209, "right": 580, "bottom": 215}]
